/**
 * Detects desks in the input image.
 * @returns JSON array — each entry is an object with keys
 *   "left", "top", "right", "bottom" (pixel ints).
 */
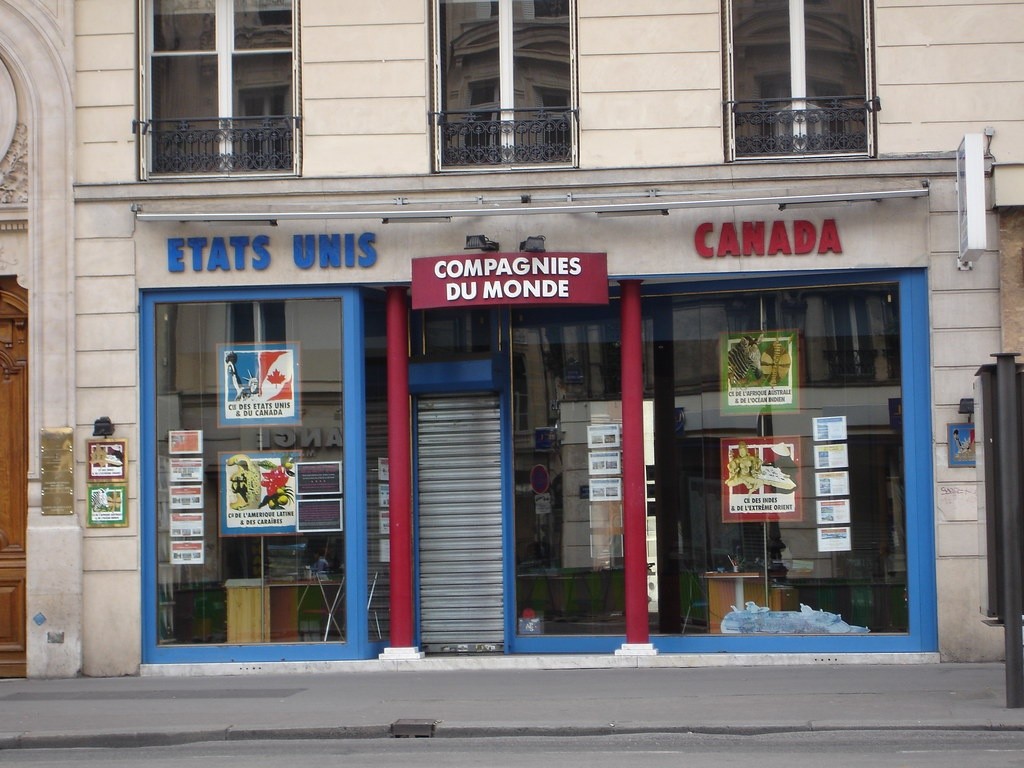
[
  {"left": 226, "top": 580, "right": 341, "bottom": 643},
  {"left": 699, "top": 571, "right": 799, "bottom": 635}
]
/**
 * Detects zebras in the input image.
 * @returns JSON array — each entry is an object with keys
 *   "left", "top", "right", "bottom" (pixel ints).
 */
[{"left": 727, "top": 333, "right": 765, "bottom": 384}]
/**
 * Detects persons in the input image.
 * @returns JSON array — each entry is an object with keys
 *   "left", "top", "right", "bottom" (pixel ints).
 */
[{"left": 550, "top": 470, "right": 595, "bottom": 620}]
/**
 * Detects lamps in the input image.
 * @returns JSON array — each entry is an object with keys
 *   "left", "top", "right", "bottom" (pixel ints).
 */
[
  {"left": 519, "top": 235, "right": 546, "bottom": 252},
  {"left": 382, "top": 217, "right": 451, "bottom": 224},
  {"left": 958, "top": 399, "right": 974, "bottom": 422},
  {"left": 93, "top": 417, "right": 115, "bottom": 438},
  {"left": 595, "top": 209, "right": 669, "bottom": 216},
  {"left": 778, "top": 198, "right": 882, "bottom": 211},
  {"left": 464, "top": 235, "right": 499, "bottom": 252},
  {"left": 179, "top": 220, "right": 278, "bottom": 226}
]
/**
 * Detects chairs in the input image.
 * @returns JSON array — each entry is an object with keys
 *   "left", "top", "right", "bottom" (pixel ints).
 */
[{"left": 682, "top": 578, "right": 704, "bottom": 633}]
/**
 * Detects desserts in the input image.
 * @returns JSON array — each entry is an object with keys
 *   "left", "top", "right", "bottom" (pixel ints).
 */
[{"left": 756, "top": 464, "right": 796, "bottom": 490}]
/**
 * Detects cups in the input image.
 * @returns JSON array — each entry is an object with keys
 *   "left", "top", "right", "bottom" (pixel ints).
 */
[{"left": 733, "top": 566, "right": 739, "bottom": 572}]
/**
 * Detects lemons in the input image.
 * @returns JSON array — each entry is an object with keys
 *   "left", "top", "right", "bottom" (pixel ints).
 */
[{"left": 268, "top": 462, "right": 293, "bottom": 507}]
